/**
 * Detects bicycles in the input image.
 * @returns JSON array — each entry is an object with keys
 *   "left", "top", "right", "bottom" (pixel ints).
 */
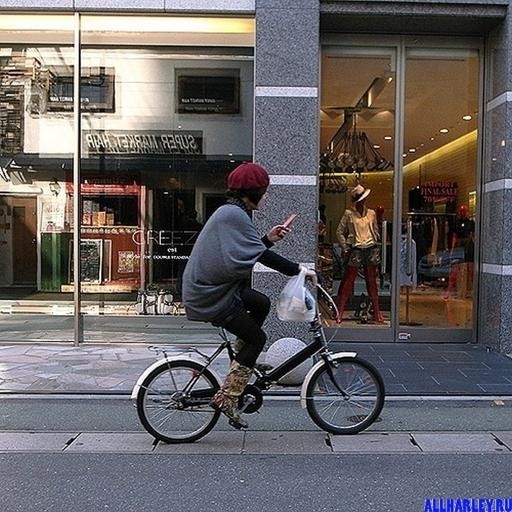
[{"left": 131, "top": 269, "right": 384, "bottom": 443}]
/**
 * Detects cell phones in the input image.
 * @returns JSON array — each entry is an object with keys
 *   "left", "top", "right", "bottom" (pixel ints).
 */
[{"left": 278, "top": 213, "right": 300, "bottom": 236}]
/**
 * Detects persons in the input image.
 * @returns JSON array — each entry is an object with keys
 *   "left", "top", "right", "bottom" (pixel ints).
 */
[
  {"left": 442, "top": 204, "right": 475, "bottom": 299},
  {"left": 333, "top": 184, "right": 390, "bottom": 325},
  {"left": 181, "top": 161, "right": 319, "bottom": 430},
  {"left": 373, "top": 203, "right": 392, "bottom": 291},
  {"left": 175, "top": 210, "right": 205, "bottom": 292}
]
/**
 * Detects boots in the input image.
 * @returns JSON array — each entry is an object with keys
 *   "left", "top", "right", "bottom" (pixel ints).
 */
[
  {"left": 231, "top": 337, "right": 271, "bottom": 371},
  {"left": 210, "top": 358, "right": 253, "bottom": 429}
]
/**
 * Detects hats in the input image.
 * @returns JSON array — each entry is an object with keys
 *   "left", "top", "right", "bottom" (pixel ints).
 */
[
  {"left": 227, "top": 161, "right": 270, "bottom": 189},
  {"left": 350, "top": 185, "right": 371, "bottom": 204}
]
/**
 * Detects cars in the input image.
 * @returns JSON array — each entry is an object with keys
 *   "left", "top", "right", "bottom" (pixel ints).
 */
[{"left": 418, "top": 247, "right": 464, "bottom": 287}]
